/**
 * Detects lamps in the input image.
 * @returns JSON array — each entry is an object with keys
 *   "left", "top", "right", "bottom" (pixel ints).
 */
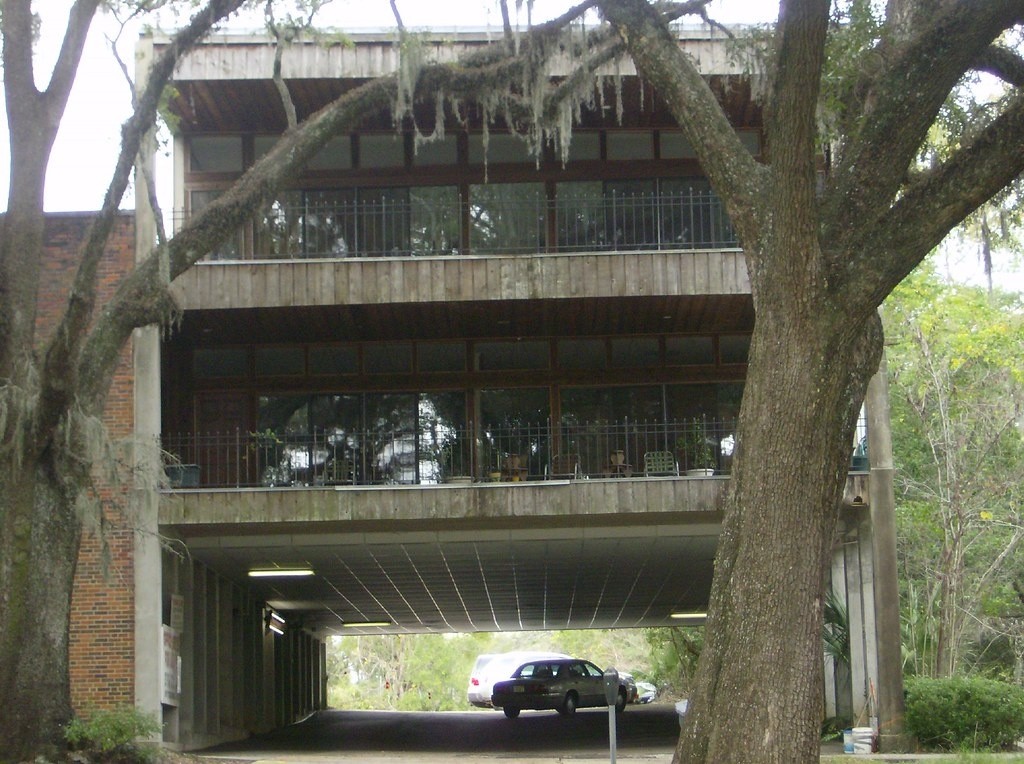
[
  {"left": 248, "top": 569, "right": 315, "bottom": 576},
  {"left": 267, "top": 624, "right": 285, "bottom": 635},
  {"left": 342, "top": 620, "right": 391, "bottom": 627},
  {"left": 669, "top": 611, "right": 708, "bottom": 619},
  {"left": 268, "top": 610, "right": 286, "bottom": 624}
]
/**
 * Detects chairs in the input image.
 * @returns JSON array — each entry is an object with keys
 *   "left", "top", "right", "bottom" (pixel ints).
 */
[
  {"left": 500, "top": 454, "right": 528, "bottom": 481},
  {"left": 320, "top": 459, "right": 358, "bottom": 486},
  {"left": 643, "top": 450, "right": 681, "bottom": 477},
  {"left": 543, "top": 453, "right": 582, "bottom": 479},
  {"left": 599, "top": 450, "right": 632, "bottom": 478}
]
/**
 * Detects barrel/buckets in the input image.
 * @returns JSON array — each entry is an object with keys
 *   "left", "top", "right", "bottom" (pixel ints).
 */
[
  {"left": 852, "top": 728, "right": 873, "bottom": 753},
  {"left": 843, "top": 730, "right": 854, "bottom": 753}
]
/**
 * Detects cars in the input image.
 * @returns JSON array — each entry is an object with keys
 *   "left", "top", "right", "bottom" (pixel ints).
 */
[
  {"left": 634, "top": 683, "right": 657, "bottom": 702},
  {"left": 491, "top": 659, "right": 632, "bottom": 717},
  {"left": 467, "top": 652, "right": 637, "bottom": 712}
]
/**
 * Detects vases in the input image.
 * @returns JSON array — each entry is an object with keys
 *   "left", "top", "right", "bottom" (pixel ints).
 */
[
  {"left": 447, "top": 476, "right": 475, "bottom": 483},
  {"left": 165, "top": 464, "right": 202, "bottom": 488},
  {"left": 853, "top": 456, "right": 870, "bottom": 470}
]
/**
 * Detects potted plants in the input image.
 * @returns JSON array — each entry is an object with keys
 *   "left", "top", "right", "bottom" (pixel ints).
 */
[{"left": 673, "top": 415, "right": 720, "bottom": 477}]
